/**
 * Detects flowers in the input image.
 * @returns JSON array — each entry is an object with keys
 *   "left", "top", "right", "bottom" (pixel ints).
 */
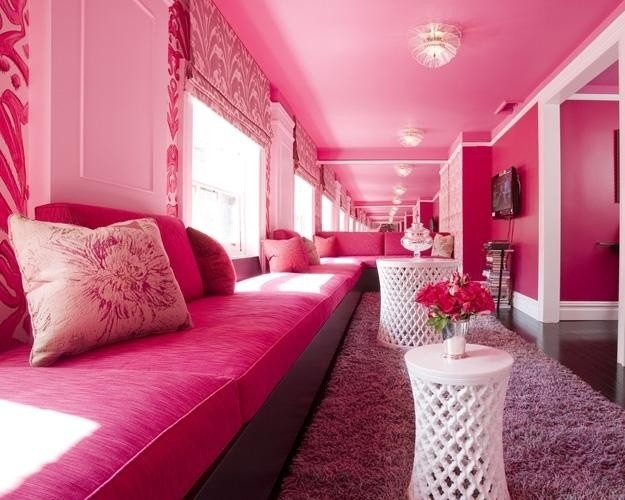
[{"left": 415, "top": 271, "right": 496, "bottom": 333}]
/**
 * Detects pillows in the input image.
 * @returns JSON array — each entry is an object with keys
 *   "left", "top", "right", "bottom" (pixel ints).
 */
[
  {"left": 313, "top": 236, "right": 337, "bottom": 257},
  {"left": 7, "top": 214, "right": 194, "bottom": 370},
  {"left": 262, "top": 236, "right": 311, "bottom": 274},
  {"left": 186, "top": 227, "right": 235, "bottom": 299},
  {"left": 303, "top": 238, "right": 320, "bottom": 267},
  {"left": 430, "top": 234, "right": 454, "bottom": 258}
]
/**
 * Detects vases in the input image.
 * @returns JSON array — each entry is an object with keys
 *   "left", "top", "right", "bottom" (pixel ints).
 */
[{"left": 446, "top": 316, "right": 466, "bottom": 355}]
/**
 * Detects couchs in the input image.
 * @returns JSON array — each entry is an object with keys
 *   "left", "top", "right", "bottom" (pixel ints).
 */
[
  {"left": 311, "top": 230, "right": 455, "bottom": 291},
  {"left": 1, "top": 227, "right": 366, "bottom": 500}
]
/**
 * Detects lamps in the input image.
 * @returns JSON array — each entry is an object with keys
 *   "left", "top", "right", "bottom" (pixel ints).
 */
[{"left": 387, "top": 23, "right": 461, "bottom": 222}]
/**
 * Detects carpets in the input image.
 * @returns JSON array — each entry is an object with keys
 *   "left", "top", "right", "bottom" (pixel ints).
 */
[{"left": 269, "top": 291, "right": 625, "bottom": 500}]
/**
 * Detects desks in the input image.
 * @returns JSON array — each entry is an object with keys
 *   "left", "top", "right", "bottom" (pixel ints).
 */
[
  {"left": 374, "top": 255, "right": 464, "bottom": 353},
  {"left": 402, "top": 342, "right": 512, "bottom": 500}
]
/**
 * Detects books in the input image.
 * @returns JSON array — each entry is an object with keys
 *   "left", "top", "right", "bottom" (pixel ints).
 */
[{"left": 480, "top": 249, "right": 513, "bottom": 308}]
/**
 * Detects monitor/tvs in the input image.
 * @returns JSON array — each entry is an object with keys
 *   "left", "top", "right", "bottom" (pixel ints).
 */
[
  {"left": 431, "top": 217, "right": 435, "bottom": 231},
  {"left": 492, "top": 166, "right": 518, "bottom": 217}
]
